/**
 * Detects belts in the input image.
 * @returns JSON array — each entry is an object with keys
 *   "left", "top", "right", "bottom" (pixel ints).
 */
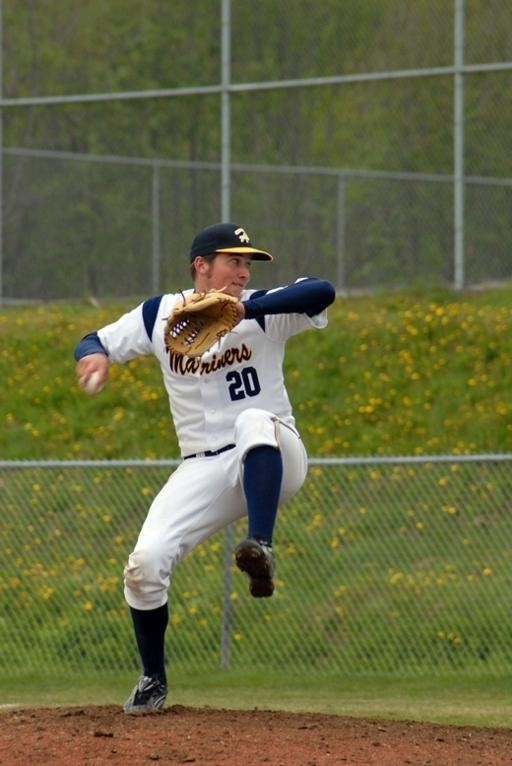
[{"left": 184, "top": 444, "right": 236, "bottom": 460}]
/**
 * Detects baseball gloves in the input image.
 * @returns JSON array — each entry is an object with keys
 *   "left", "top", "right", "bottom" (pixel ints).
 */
[{"left": 164, "top": 289, "right": 237, "bottom": 358}]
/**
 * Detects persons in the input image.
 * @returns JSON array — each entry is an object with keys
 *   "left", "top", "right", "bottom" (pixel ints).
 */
[{"left": 73, "top": 222, "right": 335, "bottom": 716}]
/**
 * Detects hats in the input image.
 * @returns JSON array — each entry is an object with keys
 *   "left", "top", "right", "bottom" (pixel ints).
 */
[{"left": 190, "top": 223, "right": 273, "bottom": 263}]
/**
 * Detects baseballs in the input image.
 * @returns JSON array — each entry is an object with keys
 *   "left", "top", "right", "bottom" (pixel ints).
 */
[{"left": 79, "top": 371, "right": 103, "bottom": 395}]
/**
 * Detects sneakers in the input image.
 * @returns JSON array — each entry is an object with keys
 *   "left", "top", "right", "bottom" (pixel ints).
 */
[
  {"left": 236, "top": 539, "right": 274, "bottom": 596},
  {"left": 124, "top": 674, "right": 166, "bottom": 716}
]
